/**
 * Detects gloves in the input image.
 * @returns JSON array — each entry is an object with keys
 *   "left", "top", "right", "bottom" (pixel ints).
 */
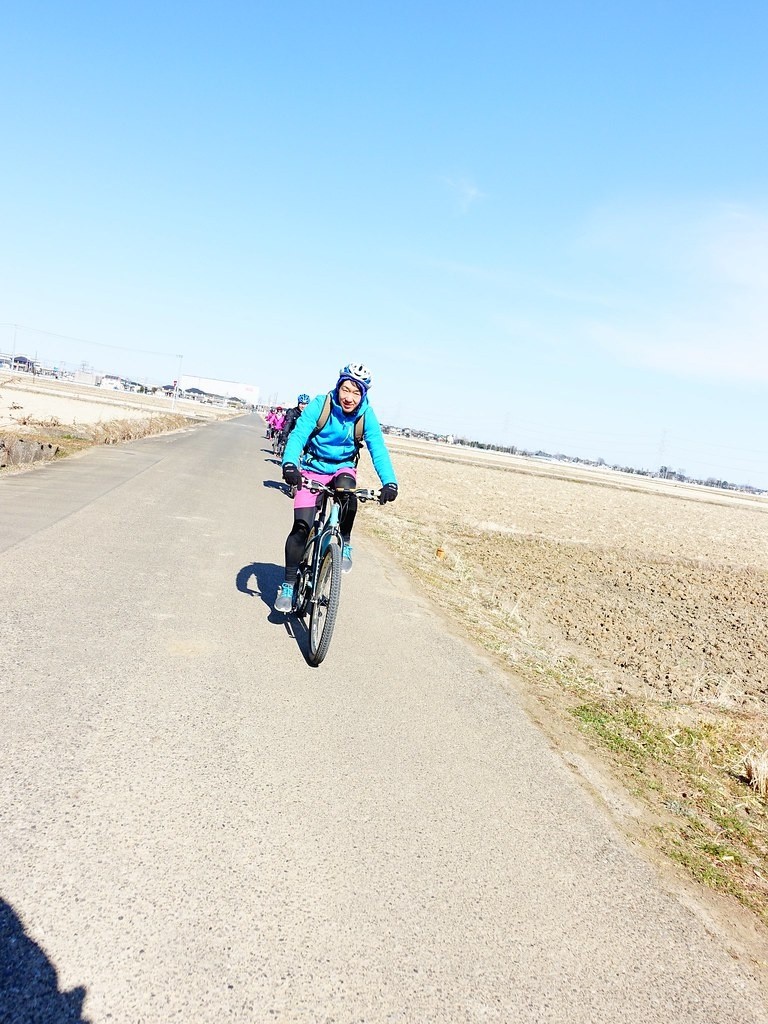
[
  {"left": 283, "top": 462, "right": 302, "bottom": 491},
  {"left": 266, "top": 418, "right": 287, "bottom": 444},
  {"left": 377, "top": 483, "right": 398, "bottom": 506}
]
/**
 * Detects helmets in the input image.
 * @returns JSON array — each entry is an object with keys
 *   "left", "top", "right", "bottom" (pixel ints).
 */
[
  {"left": 270, "top": 408, "right": 276, "bottom": 412},
  {"left": 340, "top": 362, "right": 374, "bottom": 389},
  {"left": 297, "top": 394, "right": 310, "bottom": 405},
  {"left": 277, "top": 407, "right": 282, "bottom": 410}
]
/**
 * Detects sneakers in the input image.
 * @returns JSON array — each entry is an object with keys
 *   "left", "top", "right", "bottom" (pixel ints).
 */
[
  {"left": 341, "top": 542, "right": 353, "bottom": 575},
  {"left": 274, "top": 584, "right": 293, "bottom": 613}
]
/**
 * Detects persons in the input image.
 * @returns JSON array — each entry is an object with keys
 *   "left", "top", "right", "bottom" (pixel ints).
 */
[
  {"left": 264, "top": 394, "right": 310, "bottom": 444},
  {"left": 274, "top": 363, "right": 399, "bottom": 612}
]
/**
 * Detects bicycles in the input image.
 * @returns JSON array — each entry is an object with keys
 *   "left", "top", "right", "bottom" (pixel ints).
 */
[
  {"left": 272, "top": 428, "right": 288, "bottom": 457},
  {"left": 296, "top": 475, "right": 383, "bottom": 662}
]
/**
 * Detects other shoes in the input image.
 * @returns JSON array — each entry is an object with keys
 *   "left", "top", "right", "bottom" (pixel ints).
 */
[{"left": 266, "top": 425, "right": 284, "bottom": 457}]
